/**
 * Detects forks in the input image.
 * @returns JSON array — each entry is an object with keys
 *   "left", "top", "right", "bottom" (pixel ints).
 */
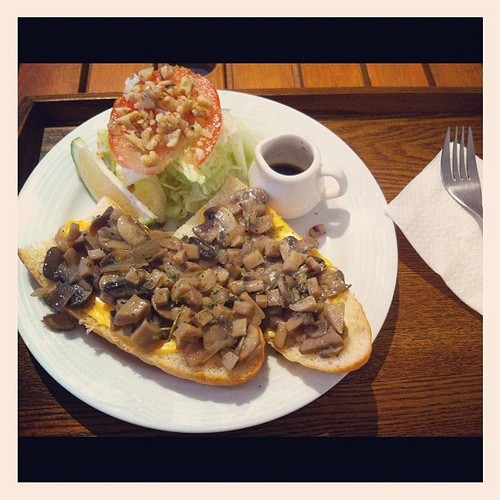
[{"left": 440, "top": 125, "right": 484, "bottom": 227}]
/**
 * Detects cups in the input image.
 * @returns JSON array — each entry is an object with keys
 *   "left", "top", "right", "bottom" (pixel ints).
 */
[{"left": 248, "top": 131, "right": 350, "bottom": 220}]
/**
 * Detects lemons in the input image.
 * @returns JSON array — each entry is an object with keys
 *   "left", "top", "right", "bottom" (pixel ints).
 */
[{"left": 71, "top": 137, "right": 167, "bottom": 228}]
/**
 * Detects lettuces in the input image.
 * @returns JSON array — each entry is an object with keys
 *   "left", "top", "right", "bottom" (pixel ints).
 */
[{"left": 97, "top": 116, "right": 255, "bottom": 229}]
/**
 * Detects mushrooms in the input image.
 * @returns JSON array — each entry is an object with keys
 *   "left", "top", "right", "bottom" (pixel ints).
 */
[{"left": 41, "top": 187, "right": 351, "bottom": 373}]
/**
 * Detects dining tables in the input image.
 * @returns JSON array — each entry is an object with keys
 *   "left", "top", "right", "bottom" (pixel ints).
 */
[{"left": 16, "top": 60, "right": 482, "bottom": 106}]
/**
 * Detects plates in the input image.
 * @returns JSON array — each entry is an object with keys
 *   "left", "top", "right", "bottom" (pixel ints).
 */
[{"left": 17, "top": 89, "right": 400, "bottom": 433}]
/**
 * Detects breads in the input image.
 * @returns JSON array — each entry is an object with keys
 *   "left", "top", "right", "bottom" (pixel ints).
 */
[
  {"left": 171, "top": 175, "right": 371, "bottom": 372},
  {"left": 16, "top": 176, "right": 264, "bottom": 384}
]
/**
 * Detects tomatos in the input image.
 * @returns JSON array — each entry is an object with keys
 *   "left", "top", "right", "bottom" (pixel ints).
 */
[{"left": 109, "top": 65, "right": 223, "bottom": 174}]
